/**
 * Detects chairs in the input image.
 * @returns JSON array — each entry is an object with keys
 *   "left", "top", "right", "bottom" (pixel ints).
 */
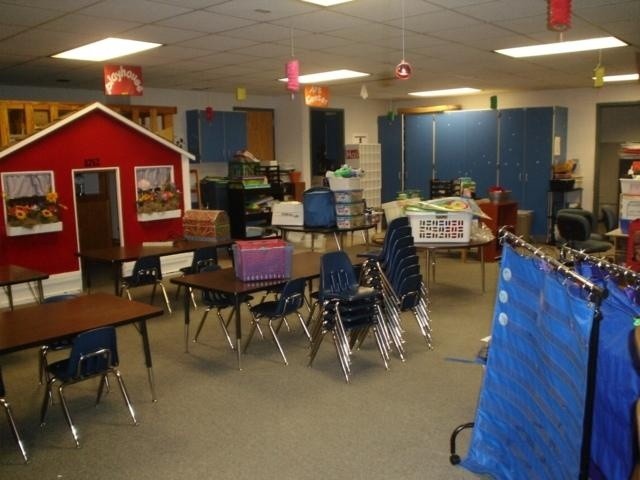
[
  {"left": 550, "top": 189, "right": 616, "bottom": 253},
  {"left": 40, "top": 293, "right": 78, "bottom": 383},
  {"left": 303, "top": 187, "right": 340, "bottom": 252},
  {"left": 39, "top": 325, "right": 139, "bottom": 449},
  {"left": 0, "top": 367, "right": 30, "bottom": 464}
]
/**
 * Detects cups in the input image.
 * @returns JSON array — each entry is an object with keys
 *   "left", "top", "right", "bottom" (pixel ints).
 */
[{"left": 620, "top": 219, "right": 631, "bottom": 234}]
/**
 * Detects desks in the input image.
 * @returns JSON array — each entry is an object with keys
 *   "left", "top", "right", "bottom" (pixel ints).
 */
[
  {"left": 76, "top": 239, "right": 234, "bottom": 296},
  {"left": 371, "top": 229, "right": 493, "bottom": 299},
  {"left": 0, "top": 261, "right": 49, "bottom": 310},
  {"left": 0, "top": 293, "right": 164, "bottom": 405},
  {"left": 605, "top": 228, "right": 629, "bottom": 262}
]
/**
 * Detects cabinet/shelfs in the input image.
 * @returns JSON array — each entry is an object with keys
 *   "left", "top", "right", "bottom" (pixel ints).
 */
[
  {"left": 186, "top": 109, "right": 247, "bottom": 164},
  {"left": 227, "top": 181, "right": 281, "bottom": 239},
  {"left": 479, "top": 201, "right": 518, "bottom": 261},
  {"left": 378, "top": 106, "right": 568, "bottom": 243}
]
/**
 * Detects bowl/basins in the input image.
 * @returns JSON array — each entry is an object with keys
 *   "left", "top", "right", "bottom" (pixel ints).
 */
[{"left": 486, "top": 191, "right": 512, "bottom": 204}]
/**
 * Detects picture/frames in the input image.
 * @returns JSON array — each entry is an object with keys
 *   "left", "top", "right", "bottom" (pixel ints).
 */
[
  {"left": 133, "top": 165, "right": 182, "bottom": 223},
  {"left": 0, "top": 170, "right": 63, "bottom": 237}
]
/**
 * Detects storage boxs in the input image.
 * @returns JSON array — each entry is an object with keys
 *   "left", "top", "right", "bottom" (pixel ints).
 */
[
  {"left": 182, "top": 209, "right": 231, "bottom": 242},
  {"left": 327, "top": 175, "right": 366, "bottom": 228}
]
